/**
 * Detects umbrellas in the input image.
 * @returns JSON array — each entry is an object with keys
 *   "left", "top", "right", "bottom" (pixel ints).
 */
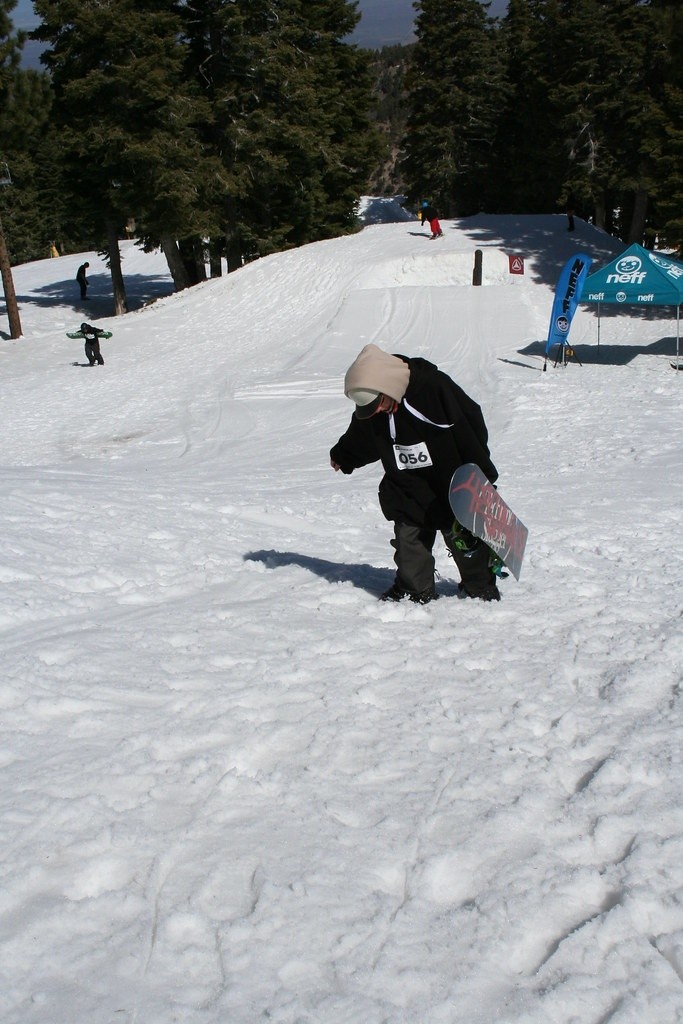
[{"left": 569, "top": 242, "right": 683, "bottom": 375}]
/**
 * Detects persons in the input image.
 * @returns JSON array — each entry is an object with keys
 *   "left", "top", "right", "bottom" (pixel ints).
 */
[
  {"left": 421, "top": 202, "right": 443, "bottom": 241},
  {"left": 329, "top": 341, "right": 508, "bottom": 604},
  {"left": 77, "top": 262, "right": 91, "bottom": 299},
  {"left": 65, "top": 323, "right": 113, "bottom": 365}
]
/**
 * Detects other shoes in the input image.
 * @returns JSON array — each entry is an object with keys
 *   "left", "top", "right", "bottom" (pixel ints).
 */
[
  {"left": 81, "top": 297, "right": 89, "bottom": 300},
  {"left": 98, "top": 362, "right": 104, "bottom": 365},
  {"left": 90, "top": 357, "right": 95, "bottom": 365},
  {"left": 431, "top": 233, "right": 443, "bottom": 239}
]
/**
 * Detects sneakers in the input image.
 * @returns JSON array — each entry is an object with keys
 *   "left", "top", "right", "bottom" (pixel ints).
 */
[
  {"left": 459, "top": 588, "right": 502, "bottom": 600},
  {"left": 378, "top": 588, "right": 436, "bottom": 604}
]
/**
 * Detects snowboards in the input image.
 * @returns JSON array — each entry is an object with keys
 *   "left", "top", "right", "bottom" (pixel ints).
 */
[
  {"left": 66, "top": 332, "right": 112, "bottom": 339},
  {"left": 448, "top": 462, "right": 529, "bottom": 582}
]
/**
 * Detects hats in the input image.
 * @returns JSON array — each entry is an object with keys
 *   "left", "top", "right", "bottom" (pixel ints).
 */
[{"left": 348, "top": 389, "right": 382, "bottom": 419}]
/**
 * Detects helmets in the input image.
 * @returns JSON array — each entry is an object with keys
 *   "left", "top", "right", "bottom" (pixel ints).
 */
[
  {"left": 80, "top": 324, "right": 88, "bottom": 330},
  {"left": 422, "top": 202, "right": 428, "bottom": 207}
]
